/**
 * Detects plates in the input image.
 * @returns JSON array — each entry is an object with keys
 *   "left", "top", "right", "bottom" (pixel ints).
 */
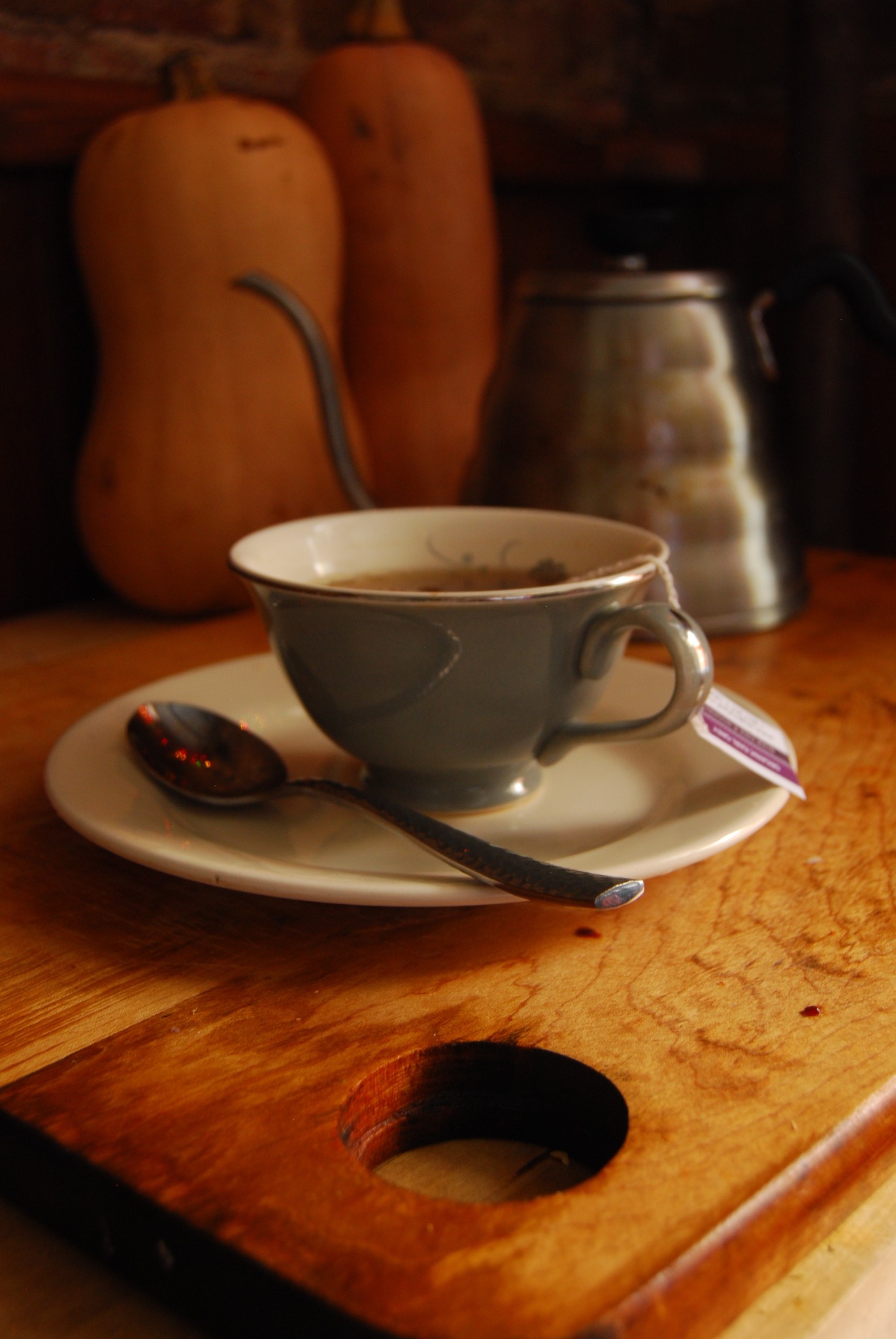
[{"left": 45, "top": 651, "right": 794, "bottom": 904}]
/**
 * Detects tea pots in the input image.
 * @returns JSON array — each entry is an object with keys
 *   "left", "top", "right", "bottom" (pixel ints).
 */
[{"left": 234, "top": 253, "right": 896, "bottom": 634}]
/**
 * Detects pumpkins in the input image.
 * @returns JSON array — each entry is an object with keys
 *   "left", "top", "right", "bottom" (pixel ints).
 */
[
  {"left": 291, "top": 11, "right": 502, "bottom": 509},
  {"left": 61, "top": 46, "right": 373, "bottom": 615}
]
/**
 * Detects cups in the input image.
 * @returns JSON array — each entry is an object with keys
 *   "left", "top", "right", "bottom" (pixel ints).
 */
[{"left": 226, "top": 506, "right": 716, "bottom": 811}]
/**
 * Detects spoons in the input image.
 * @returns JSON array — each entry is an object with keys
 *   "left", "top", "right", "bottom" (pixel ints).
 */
[{"left": 125, "top": 700, "right": 644, "bottom": 908}]
